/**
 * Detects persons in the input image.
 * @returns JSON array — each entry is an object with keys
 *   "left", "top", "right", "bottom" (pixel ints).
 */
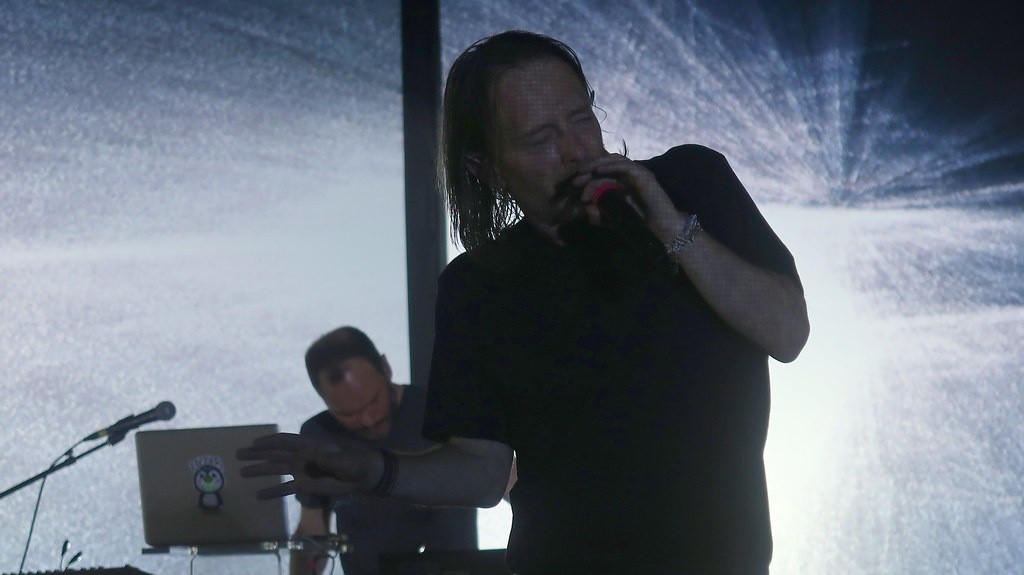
[
  {"left": 235, "top": 29, "right": 810, "bottom": 574},
  {"left": 288, "top": 326, "right": 519, "bottom": 575}
]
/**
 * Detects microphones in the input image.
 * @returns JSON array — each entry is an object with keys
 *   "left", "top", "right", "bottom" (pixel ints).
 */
[
  {"left": 83, "top": 401, "right": 176, "bottom": 441},
  {"left": 577, "top": 174, "right": 681, "bottom": 288}
]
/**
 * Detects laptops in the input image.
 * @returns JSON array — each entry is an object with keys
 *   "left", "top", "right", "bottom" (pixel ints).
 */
[{"left": 132, "top": 423, "right": 346, "bottom": 544}]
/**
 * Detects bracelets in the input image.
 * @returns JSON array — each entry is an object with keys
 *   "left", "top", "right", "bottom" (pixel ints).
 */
[
  {"left": 367, "top": 447, "right": 400, "bottom": 499},
  {"left": 664, "top": 213, "right": 703, "bottom": 256}
]
